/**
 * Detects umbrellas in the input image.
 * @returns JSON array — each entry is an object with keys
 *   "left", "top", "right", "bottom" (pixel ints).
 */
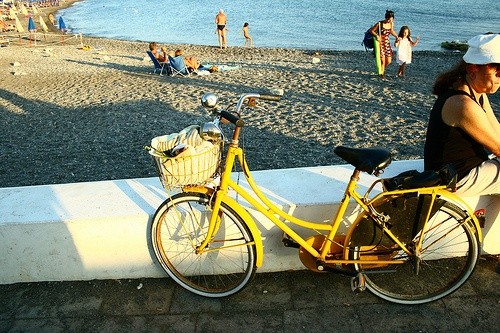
[{"left": 1, "top": 0, "right": 67, "bottom": 47}]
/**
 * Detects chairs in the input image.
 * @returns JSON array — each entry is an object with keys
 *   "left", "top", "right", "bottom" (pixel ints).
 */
[
  {"left": 146, "top": 50, "right": 171, "bottom": 75},
  {"left": 167, "top": 53, "right": 199, "bottom": 77}
]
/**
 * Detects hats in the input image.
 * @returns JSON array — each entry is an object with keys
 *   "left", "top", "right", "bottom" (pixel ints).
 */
[{"left": 462, "top": 34, "right": 500, "bottom": 64}]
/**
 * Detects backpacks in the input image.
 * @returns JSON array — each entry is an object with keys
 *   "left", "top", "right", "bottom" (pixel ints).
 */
[{"left": 361, "top": 25, "right": 374, "bottom": 53}]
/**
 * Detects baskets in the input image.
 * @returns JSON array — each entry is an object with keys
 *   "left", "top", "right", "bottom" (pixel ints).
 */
[{"left": 154, "top": 143, "right": 222, "bottom": 189}]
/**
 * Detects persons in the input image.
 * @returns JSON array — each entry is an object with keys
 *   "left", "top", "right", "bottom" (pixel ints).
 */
[
  {"left": 241, "top": 21, "right": 252, "bottom": 48},
  {"left": 422, "top": 33, "right": 499, "bottom": 199},
  {"left": 370, "top": 9, "right": 399, "bottom": 81},
  {"left": 215, "top": 8, "right": 227, "bottom": 49},
  {"left": 149, "top": 41, "right": 170, "bottom": 63},
  {"left": 175, "top": 49, "right": 201, "bottom": 73},
  {"left": 393, "top": 25, "right": 420, "bottom": 77}
]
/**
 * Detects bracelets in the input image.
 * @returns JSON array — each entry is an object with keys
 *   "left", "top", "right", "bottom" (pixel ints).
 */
[
  {"left": 376, "top": 35, "right": 379, "bottom": 38},
  {"left": 163, "top": 51, "right": 166, "bottom": 52},
  {"left": 395, "top": 35, "right": 397, "bottom": 37}
]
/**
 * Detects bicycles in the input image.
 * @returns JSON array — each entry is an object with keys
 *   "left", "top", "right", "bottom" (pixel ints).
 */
[{"left": 148, "top": 91, "right": 487, "bottom": 305}]
